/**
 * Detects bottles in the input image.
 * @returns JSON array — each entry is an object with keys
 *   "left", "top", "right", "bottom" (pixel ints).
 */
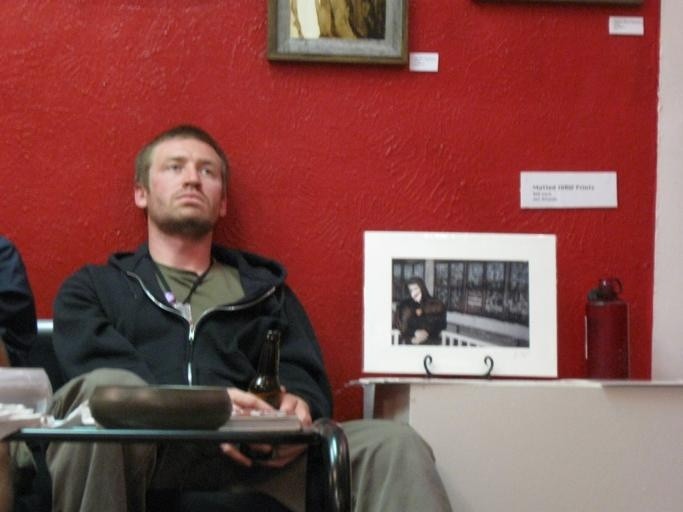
[
  {"left": 238, "top": 323, "right": 281, "bottom": 459},
  {"left": 582, "top": 276, "right": 630, "bottom": 380}
]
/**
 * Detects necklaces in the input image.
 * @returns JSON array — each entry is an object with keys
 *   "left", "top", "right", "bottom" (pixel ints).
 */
[{"left": 149, "top": 254, "right": 216, "bottom": 324}]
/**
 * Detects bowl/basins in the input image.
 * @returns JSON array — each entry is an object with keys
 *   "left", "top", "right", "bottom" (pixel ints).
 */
[
  {"left": 0, "top": 365, "right": 52, "bottom": 421},
  {"left": 89, "top": 384, "right": 234, "bottom": 432}
]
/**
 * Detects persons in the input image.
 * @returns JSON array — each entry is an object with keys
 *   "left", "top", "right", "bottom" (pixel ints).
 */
[
  {"left": 48, "top": 122, "right": 455, "bottom": 510},
  {"left": 391, "top": 277, "right": 448, "bottom": 345},
  {"left": 1, "top": 231, "right": 44, "bottom": 510}
]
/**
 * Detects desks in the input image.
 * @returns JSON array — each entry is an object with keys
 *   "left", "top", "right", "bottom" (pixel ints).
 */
[{"left": 0, "top": 414, "right": 350, "bottom": 511}]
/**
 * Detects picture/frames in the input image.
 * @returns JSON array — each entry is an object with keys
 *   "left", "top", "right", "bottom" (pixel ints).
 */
[
  {"left": 361, "top": 231, "right": 558, "bottom": 379},
  {"left": 267, "top": 0, "right": 408, "bottom": 66}
]
